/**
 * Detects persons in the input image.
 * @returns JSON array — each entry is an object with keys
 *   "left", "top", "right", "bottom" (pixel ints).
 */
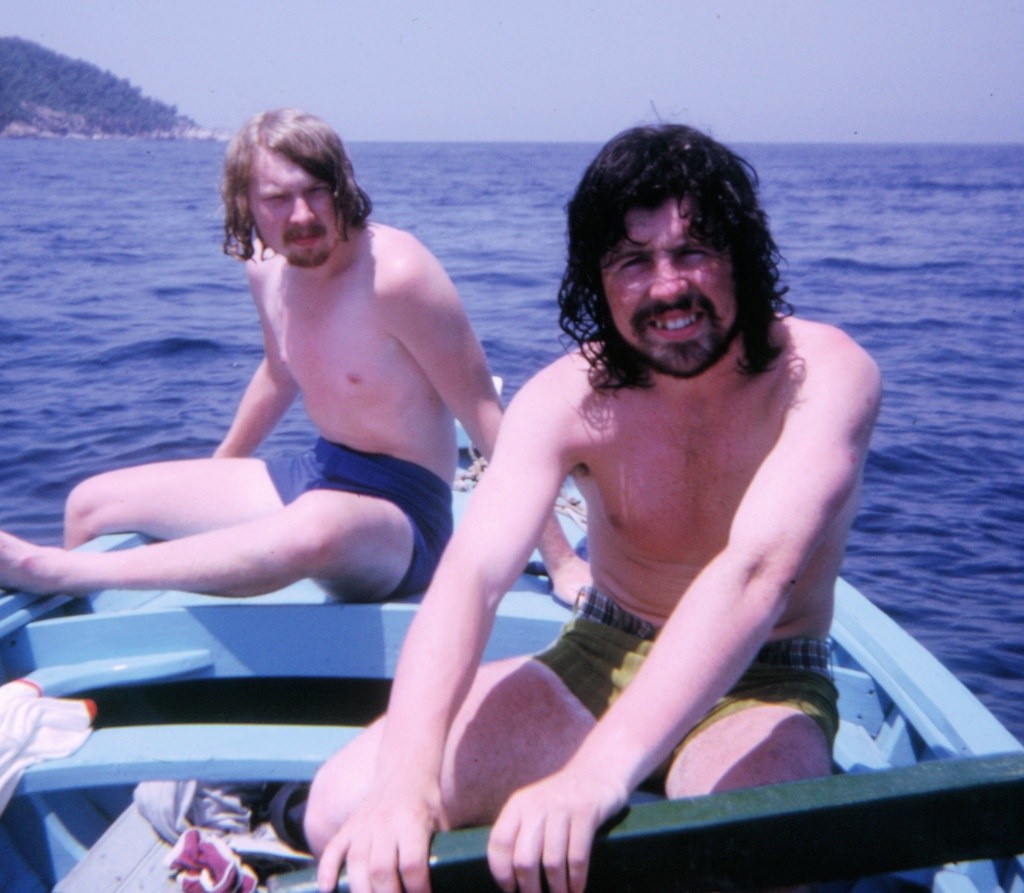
[
  {"left": 0, "top": 109, "right": 595, "bottom": 608},
  {"left": 304, "top": 124, "right": 882, "bottom": 893}
]
